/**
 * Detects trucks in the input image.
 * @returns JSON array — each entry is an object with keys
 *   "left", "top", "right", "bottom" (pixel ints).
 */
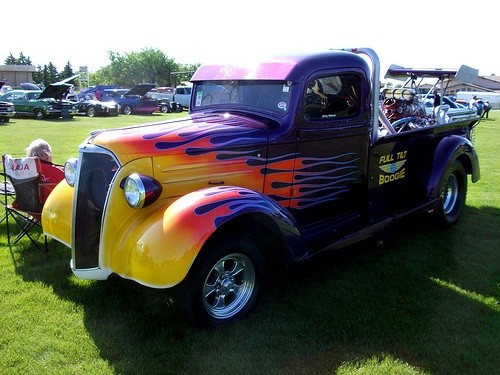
[{"left": 41, "top": 48, "right": 482, "bottom": 332}]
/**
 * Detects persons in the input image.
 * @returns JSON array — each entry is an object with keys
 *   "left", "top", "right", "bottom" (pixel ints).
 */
[
  {"left": 12, "top": 138, "right": 65, "bottom": 209},
  {"left": 470, "top": 96, "right": 492, "bottom": 118},
  {"left": 95, "top": 89, "right": 103, "bottom": 102},
  {"left": 433, "top": 90, "right": 440, "bottom": 119}
]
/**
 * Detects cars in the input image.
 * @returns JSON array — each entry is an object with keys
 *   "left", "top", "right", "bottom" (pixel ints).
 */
[
  {"left": 0, "top": 101, "right": 16, "bottom": 124},
  {"left": 0, "top": 83, "right": 80, "bottom": 119},
  {"left": 92, "top": 84, "right": 161, "bottom": 115},
  {"left": 173, "top": 86, "right": 192, "bottom": 112},
  {"left": 145, "top": 88, "right": 178, "bottom": 111},
  {"left": 19, "top": 83, "right": 41, "bottom": 89},
  {"left": 71, "top": 97, "right": 121, "bottom": 118}
]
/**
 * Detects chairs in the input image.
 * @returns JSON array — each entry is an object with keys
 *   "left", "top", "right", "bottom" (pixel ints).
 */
[
  {"left": 0, "top": 154, "right": 48, "bottom": 251},
  {"left": 304, "top": 80, "right": 358, "bottom": 118}
]
